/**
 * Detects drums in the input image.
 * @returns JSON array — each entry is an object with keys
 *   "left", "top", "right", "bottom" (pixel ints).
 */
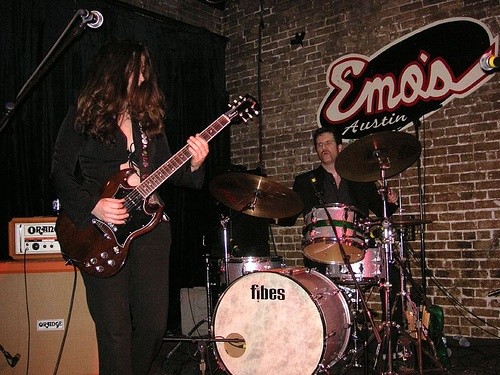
[
  {"left": 218, "top": 255, "right": 284, "bottom": 291},
  {"left": 209, "top": 265, "right": 351, "bottom": 375},
  {"left": 300, "top": 202, "right": 366, "bottom": 263},
  {"left": 325, "top": 243, "right": 386, "bottom": 285}
]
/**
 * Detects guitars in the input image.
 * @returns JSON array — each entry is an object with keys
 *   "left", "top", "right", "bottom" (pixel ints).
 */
[{"left": 54, "top": 92, "right": 261, "bottom": 277}]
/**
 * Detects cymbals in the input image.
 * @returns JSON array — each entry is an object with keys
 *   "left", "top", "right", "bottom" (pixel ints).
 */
[
  {"left": 209, "top": 171, "right": 304, "bottom": 219},
  {"left": 335, "top": 131, "right": 421, "bottom": 181},
  {"left": 364, "top": 214, "right": 432, "bottom": 227}
]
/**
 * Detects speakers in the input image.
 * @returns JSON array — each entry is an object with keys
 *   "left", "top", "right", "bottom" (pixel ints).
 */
[{"left": 0, "top": 260, "right": 99, "bottom": 375}]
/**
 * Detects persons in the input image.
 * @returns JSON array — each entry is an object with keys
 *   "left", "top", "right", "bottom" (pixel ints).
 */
[
  {"left": 48, "top": 37, "right": 210, "bottom": 375},
  {"left": 273, "top": 126, "right": 415, "bottom": 361}
]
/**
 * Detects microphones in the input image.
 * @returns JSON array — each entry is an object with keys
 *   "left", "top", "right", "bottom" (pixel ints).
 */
[
  {"left": 370, "top": 227, "right": 384, "bottom": 239},
  {"left": 11, "top": 354, "right": 21, "bottom": 367},
  {"left": 310, "top": 174, "right": 322, "bottom": 192},
  {"left": 78, "top": 10, "right": 104, "bottom": 29}
]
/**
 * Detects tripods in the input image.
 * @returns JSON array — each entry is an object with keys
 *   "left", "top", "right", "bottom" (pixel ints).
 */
[{"left": 317, "top": 117, "right": 453, "bottom": 375}]
